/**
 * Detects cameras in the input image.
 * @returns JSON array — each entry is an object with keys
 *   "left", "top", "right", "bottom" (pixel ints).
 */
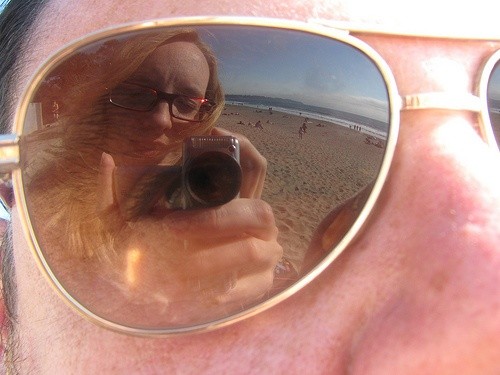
[{"left": 113, "top": 135, "right": 242, "bottom": 219}]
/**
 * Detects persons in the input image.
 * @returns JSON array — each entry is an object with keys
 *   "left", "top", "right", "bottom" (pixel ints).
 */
[
  {"left": 223, "top": 102, "right": 278, "bottom": 128},
  {"left": 26, "top": 27, "right": 284, "bottom": 329},
  {"left": 299, "top": 112, "right": 327, "bottom": 139},
  {"left": 1, "top": 0, "right": 500, "bottom": 374},
  {"left": 350, "top": 125, "right": 362, "bottom": 131}
]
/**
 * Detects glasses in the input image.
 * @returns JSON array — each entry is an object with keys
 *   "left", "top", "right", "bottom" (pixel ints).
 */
[
  {"left": 106, "top": 80, "right": 208, "bottom": 123},
  {"left": 0, "top": 16, "right": 500, "bottom": 338}
]
